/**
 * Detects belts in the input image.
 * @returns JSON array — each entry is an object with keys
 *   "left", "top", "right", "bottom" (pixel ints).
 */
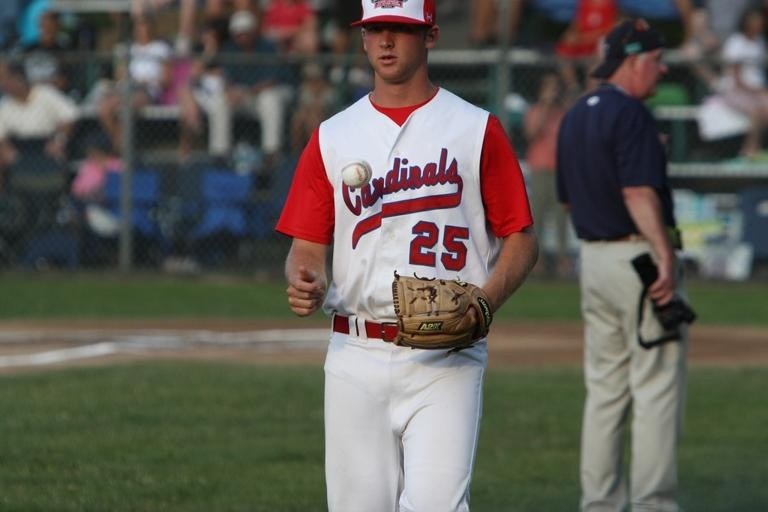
[{"left": 333, "top": 314, "right": 399, "bottom": 341}]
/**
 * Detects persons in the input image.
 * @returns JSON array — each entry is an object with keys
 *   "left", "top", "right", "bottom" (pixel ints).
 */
[
  {"left": 553, "top": 11, "right": 692, "bottom": 511},
  {"left": 467, "top": 0, "right": 768, "bottom": 287},
  {"left": 272, "top": 0, "right": 542, "bottom": 511},
  {"left": 0, "top": 0, "right": 374, "bottom": 278}
]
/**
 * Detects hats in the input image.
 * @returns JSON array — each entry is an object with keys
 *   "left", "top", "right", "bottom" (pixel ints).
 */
[
  {"left": 351, "top": 1, "right": 436, "bottom": 26},
  {"left": 589, "top": 20, "right": 664, "bottom": 79}
]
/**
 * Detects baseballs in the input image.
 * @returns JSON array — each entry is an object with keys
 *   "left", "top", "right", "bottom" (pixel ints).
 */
[{"left": 343, "top": 160, "right": 371, "bottom": 189}]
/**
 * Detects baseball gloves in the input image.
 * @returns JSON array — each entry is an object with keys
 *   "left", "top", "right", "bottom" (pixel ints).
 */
[{"left": 392, "top": 273, "right": 495, "bottom": 348}]
